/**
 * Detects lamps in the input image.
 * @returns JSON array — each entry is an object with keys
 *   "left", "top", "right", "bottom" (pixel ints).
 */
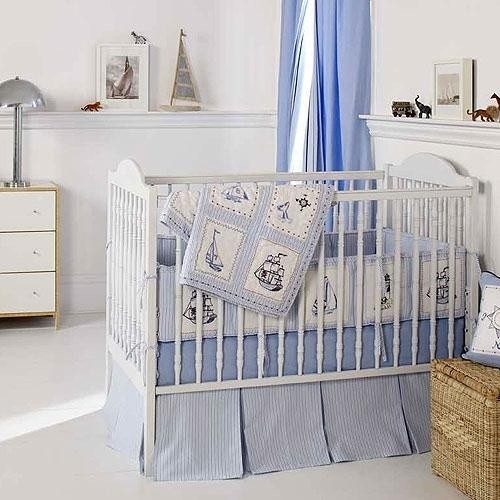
[{"left": 0, "top": 75, "right": 46, "bottom": 188}]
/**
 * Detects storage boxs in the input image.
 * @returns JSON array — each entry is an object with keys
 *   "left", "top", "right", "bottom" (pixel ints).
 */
[{"left": 427, "top": 355, "right": 500, "bottom": 499}]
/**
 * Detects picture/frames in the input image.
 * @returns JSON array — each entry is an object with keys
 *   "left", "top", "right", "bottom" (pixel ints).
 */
[
  {"left": 94, "top": 43, "right": 150, "bottom": 112},
  {"left": 431, "top": 57, "right": 473, "bottom": 119}
]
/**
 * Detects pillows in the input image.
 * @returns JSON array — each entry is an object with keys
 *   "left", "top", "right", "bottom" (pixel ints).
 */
[{"left": 461, "top": 268, "right": 500, "bottom": 368}]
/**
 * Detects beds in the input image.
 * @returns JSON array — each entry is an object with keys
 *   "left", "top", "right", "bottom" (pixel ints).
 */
[{"left": 102, "top": 152, "right": 482, "bottom": 485}]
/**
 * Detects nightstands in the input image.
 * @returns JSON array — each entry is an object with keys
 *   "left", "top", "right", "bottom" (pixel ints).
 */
[{"left": 0, "top": 179, "right": 61, "bottom": 329}]
